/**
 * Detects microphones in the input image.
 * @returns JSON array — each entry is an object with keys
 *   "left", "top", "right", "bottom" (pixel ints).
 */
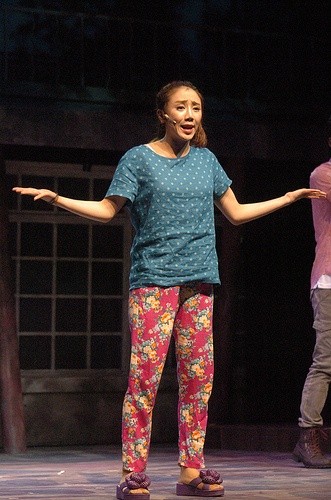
[{"left": 162, "top": 113, "right": 176, "bottom": 124}]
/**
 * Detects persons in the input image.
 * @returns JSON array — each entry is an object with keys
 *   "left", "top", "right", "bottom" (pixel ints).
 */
[
  {"left": 294, "top": 151, "right": 331, "bottom": 467},
  {"left": 10, "top": 77, "right": 328, "bottom": 498}
]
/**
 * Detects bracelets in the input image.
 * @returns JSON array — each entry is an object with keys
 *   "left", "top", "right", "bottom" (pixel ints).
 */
[{"left": 46, "top": 194, "right": 58, "bottom": 207}]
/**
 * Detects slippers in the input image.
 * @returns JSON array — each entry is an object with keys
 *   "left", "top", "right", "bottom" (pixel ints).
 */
[
  {"left": 174, "top": 469, "right": 228, "bottom": 496},
  {"left": 116, "top": 474, "right": 151, "bottom": 500}
]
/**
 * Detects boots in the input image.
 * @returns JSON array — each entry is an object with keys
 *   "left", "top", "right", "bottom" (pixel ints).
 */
[{"left": 292, "top": 425, "right": 331, "bottom": 469}]
